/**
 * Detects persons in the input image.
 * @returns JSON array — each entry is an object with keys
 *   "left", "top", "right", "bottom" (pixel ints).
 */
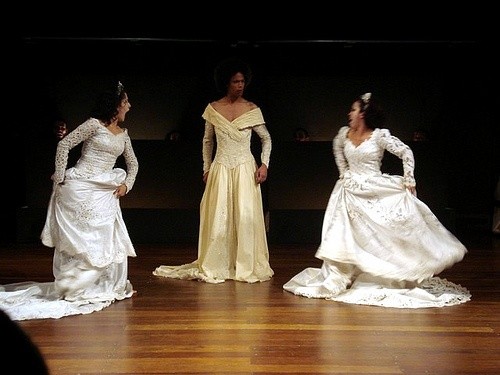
[
  {"left": 201, "top": 66, "right": 273, "bottom": 275},
  {"left": 412, "top": 129, "right": 429, "bottom": 141},
  {"left": 166, "top": 130, "right": 185, "bottom": 140},
  {"left": 293, "top": 128, "right": 311, "bottom": 141},
  {"left": 49, "top": 82, "right": 140, "bottom": 301},
  {"left": 50, "top": 120, "right": 70, "bottom": 141},
  {"left": 332, "top": 92, "right": 416, "bottom": 297}
]
